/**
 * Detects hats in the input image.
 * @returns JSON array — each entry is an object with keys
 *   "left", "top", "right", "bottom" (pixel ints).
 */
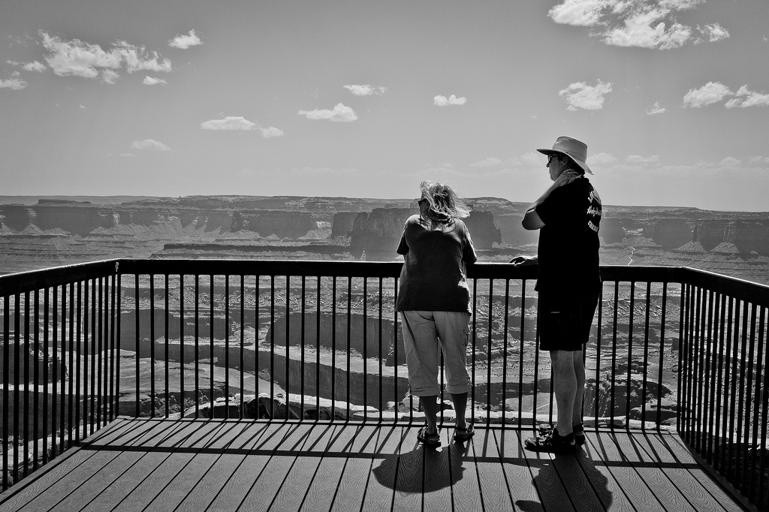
[{"left": 536, "top": 136, "right": 595, "bottom": 175}]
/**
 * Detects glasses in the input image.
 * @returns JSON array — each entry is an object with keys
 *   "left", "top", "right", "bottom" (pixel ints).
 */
[{"left": 548, "top": 154, "right": 558, "bottom": 163}]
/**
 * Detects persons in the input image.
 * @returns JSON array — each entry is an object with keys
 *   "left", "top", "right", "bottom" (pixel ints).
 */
[
  {"left": 395, "top": 180, "right": 478, "bottom": 447},
  {"left": 509, "top": 137, "right": 601, "bottom": 451}
]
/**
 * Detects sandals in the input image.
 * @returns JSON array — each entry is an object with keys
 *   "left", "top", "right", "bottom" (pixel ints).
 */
[
  {"left": 525, "top": 424, "right": 585, "bottom": 454},
  {"left": 454, "top": 425, "right": 474, "bottom": 442},
  {"left": 417, "top": 428, "right": 441, "bottom": 446}
]
